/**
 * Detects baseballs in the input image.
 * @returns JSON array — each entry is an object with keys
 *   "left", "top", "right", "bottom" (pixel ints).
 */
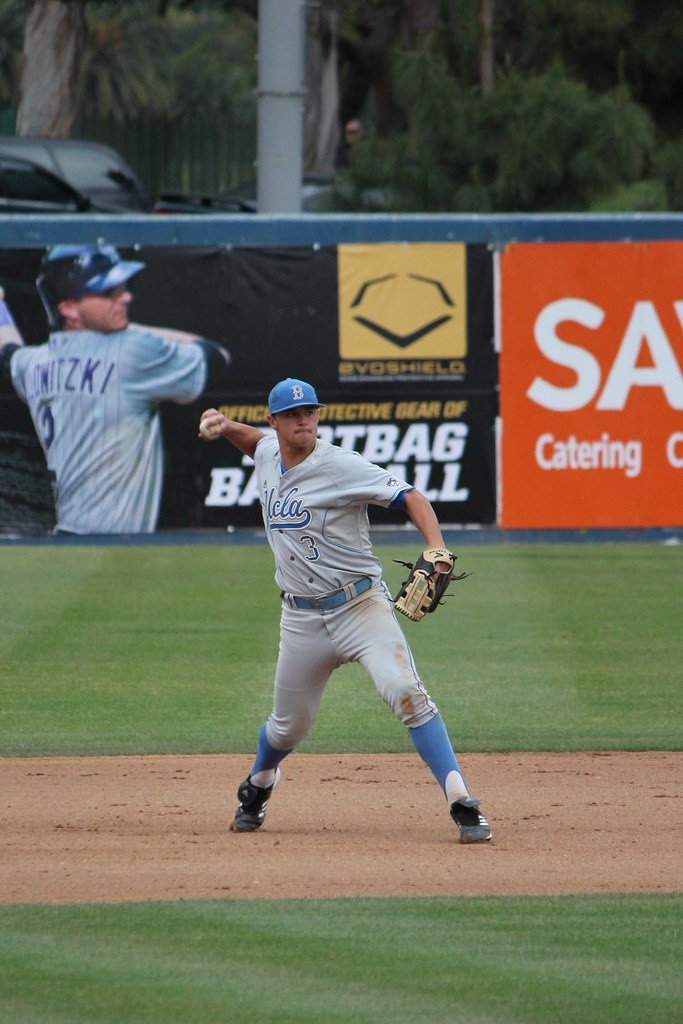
[{"left": 198, "top": 418, "right": 221, "bottom": 440}]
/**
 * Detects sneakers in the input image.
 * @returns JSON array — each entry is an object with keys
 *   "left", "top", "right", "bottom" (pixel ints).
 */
[
  {"left": 449, "top": 796, "right": 492, "bottom": 841},
  {"left": 232, "top": 767, "right": 280, "bottom": 832}
]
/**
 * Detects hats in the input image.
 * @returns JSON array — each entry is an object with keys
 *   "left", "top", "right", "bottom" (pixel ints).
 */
[
  {"left": 269, "top": 378, "right": 327, "bottom": 416},
  {"left": 44, "top": 243, "right": 147, "bottom": 298}
]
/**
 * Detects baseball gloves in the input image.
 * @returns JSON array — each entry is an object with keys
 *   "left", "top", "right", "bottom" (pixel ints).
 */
[{"left": 383, "top": 550, "right": 473, "bottom": 618}]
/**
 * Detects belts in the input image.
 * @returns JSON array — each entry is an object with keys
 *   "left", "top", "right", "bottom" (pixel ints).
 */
[{"left": 280, "top": 578, "right": 371, "bottom": 610}]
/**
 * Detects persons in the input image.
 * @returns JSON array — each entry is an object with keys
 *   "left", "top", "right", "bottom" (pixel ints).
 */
[
  {"left": 0, "top": 244, "right": 232, "bottom": 539},
  {"left": 199, "top": 379, "right": 496, "bottom": 845}
]
